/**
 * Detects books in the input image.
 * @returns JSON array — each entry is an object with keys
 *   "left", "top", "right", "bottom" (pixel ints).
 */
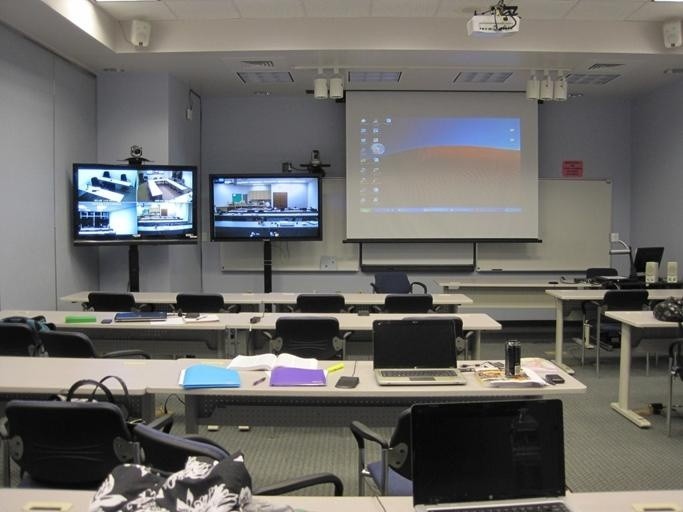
[
  {"left": 177, "top": 365, "right": 241, "bottom": 389},
  {"left": 476, "top": 367, "right": 547, "bottom": 388},
  {"left": 227, "top": 353, "right": 320, "bottom": 370},
  {"left": 183, "top": 313, "right": 220, "bottom": 324}
]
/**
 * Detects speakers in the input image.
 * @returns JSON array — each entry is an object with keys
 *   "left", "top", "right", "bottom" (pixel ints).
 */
[
  {"left": 646, "top": 262, "right": 658, "bottom": 283},
  {"left": 663, "top": 21, "right": 682, "bottom": 48},
  {"left": 131, "top": 20, "right": 151, "bottom": 45},
  {"left": 667, "top": 262, "right": 678, "bottom": 283}
]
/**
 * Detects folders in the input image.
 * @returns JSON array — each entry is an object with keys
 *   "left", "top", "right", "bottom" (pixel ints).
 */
[
  {"left": 177, "top": 364, "right": 240, "bottom": 388},
  {"left": 268, "top": 366, "right": 328, "bottom": 386}
]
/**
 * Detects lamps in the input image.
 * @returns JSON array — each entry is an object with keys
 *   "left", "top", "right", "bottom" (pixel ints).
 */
[{"left": 292, "top": 64, "right": 572, "bottom": 101}]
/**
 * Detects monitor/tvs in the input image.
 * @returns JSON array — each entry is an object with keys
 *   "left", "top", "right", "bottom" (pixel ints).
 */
[
  {"left": 209, "top": 173, "right": 322, "bottom": 241},
  {"left": 634, "top": 247, "right": 664, "bottom": 280},
  {"left": 72, "top": 163, "right": 198, "bottom": 244}
]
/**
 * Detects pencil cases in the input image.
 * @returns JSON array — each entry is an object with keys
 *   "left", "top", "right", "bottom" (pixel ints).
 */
[{"left": 65, "top": 315, "right": 96, "bottom": 322}]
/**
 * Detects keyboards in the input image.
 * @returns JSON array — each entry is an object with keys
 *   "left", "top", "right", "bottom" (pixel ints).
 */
[{"left": 596, "top": 276, "right": 628, "bottom": 279}]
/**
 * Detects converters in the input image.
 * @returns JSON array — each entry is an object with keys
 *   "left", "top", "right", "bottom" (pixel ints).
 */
[{"left": 648, "top": 403, "right": 663, "bottom": 408}]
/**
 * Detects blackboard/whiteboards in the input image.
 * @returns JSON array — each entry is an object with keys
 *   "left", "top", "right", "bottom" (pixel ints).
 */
[{"left": 219, "top": 172, "right": 613, "bottom": 275}]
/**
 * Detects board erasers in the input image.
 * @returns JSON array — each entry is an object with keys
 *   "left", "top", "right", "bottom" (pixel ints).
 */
[{"left": 492, "top": 269, "right": 502, "bottom": 271}]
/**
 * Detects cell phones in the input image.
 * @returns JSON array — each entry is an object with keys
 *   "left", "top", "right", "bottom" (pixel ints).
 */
[
  {"left": 336, "top": 377, "right": 359, "bottom": 388},
  {"left": 549, "top": 282, "right": 558, "bottom": 284},
  {"left": 547, "top": 375, "right": 564, "bottom": 383},
  {"left": 101, "top": 320, "right": 112, "bottom": 323}
]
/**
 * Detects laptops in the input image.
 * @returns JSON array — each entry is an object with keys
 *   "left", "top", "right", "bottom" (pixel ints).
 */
[
  {"left": 373, "top": 320, "right": 467, "bottom": 385},
  {"left": 411, "top": 399, "right": 571, "bottom": 512},
  {"left": 115, "top": 311, "right": 166, "bottom": 322}
]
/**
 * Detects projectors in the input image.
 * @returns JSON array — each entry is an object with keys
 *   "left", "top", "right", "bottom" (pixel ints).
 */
[{"left": 467, "top": 16, "right": 519, "bottom": 38}]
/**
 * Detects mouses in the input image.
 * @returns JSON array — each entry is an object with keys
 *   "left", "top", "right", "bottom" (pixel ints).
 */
[{"left": 250, "top": 317, "right": 260, "bottom": 323}]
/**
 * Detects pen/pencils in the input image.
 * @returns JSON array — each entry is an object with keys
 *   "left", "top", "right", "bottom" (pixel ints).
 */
[
  {"left": 253, "top": 377, "right": 265, "bottom": 385},
  {"left": 544, "top": 378, "right": 555, "bottom": 385},
  {"left": 29, "top": 507, "right": 60, "bottom": 511},
  {"left": 462, "top": 364, "right": 479, "bottom": 367},
  {"left": 195, "top": 316, "right": 207, "bottom": 320}
]
